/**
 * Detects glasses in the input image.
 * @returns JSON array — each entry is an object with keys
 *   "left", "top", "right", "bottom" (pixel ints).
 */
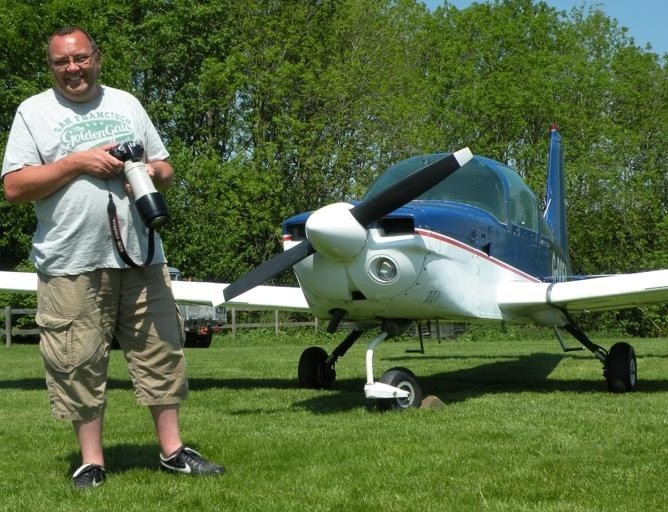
[{"left": 48, "top": 51, "right": 97, "bottom": 70}]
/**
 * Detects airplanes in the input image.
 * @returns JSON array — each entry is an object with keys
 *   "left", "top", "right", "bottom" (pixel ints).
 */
[{"left": 0, "top": 119, "right": 667, "bottom": 414}]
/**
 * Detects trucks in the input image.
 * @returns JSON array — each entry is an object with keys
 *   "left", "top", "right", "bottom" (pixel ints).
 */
[{"left": 158, "top": 266, "right": 228, "bottom": 347}]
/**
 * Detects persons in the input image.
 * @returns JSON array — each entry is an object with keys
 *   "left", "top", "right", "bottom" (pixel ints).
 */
[{"left": 0, "top": 25, "right": 226, "bottom": 489}]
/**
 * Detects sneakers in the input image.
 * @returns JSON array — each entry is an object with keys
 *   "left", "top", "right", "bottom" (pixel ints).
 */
[
  {"left": 72, "top": 463, "right": 106, "bottom": 494},
  {"left": 159, "top": 443, "right": 225, "bottom": 477}
]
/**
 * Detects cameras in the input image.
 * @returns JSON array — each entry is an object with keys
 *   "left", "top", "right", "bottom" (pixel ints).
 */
[{"left": 108, "top": 138, "right": 172, "bottom": 228}]
